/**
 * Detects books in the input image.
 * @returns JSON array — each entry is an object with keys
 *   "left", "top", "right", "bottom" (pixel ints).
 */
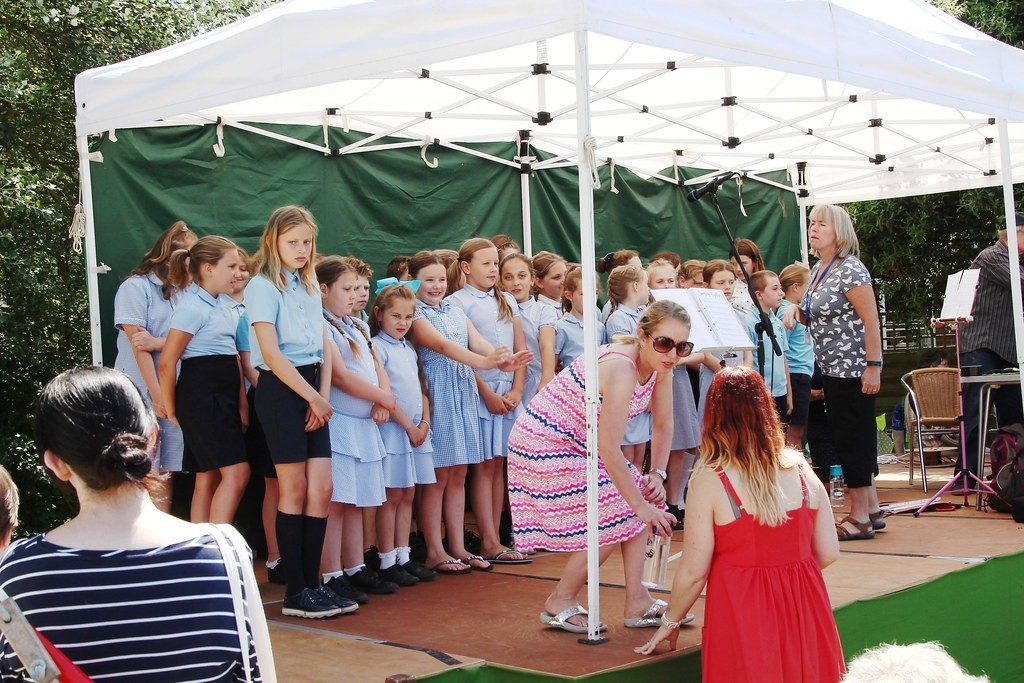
[
  {"left": 941, "top": 266, "right": 981, "bottom": 321},
  {"left": 648, "top": 286, "right": 754, "bottom": 353}
]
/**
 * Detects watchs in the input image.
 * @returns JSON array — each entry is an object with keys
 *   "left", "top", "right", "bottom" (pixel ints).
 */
[{"left": 649, "top": 468, "right": 667, "bottom": 480}]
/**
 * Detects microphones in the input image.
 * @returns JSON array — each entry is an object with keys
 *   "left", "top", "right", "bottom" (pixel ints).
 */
[{"left": 686, "top": 171, "right": 737, "bottom": 202}]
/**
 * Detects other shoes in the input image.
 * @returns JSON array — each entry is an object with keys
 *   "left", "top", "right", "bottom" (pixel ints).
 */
[
  {"left": 952, "top": 481, "right": 976, "bottom": 496},
  {"left": 665, "top": 501, "right": 685, "bottom": 530},
  {"left": 268, "top": 528, "right": 537, "bottom": 618}
]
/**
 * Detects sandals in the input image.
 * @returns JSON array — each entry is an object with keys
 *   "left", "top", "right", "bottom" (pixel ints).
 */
[
  {"left": 836, "top": 517, "right": 875, "bottom": 539},
  {"left": 869, "top": 510, "right": 886, "bottom": 529}
]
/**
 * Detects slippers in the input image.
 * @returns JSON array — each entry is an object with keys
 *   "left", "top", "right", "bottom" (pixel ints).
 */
[
  {"left": 625, "top": 599, "right": 695, "bottom": 627},
  {"left": 539, "top": 605, "right": 607, "bottom": 632}
]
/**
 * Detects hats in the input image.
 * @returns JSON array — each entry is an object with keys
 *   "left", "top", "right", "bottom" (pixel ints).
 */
[{"left": 999, "top": 212, "right": 1024, "bottom": 231}]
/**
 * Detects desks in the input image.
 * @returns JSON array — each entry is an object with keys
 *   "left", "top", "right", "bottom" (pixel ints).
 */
[{"left": 959, "top": 372, "right": 1021, "bottom": 513}]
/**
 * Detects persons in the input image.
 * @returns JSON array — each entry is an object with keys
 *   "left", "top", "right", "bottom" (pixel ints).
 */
[
  {"left": 950, "top": 212, "right": 1023, "bottom": 495},
  {"left": 919, "top": 349, "right": 949, "bottom": 369},
  {"left": 0, "top": 464, "right": 20, "bottom": 560},
  {"left": 891, "top": 400, "right": 959, "bottom": 453},
  {"left": 634, "top": 364, "right": 848, "bottom": 683},
  {"left": 506, "top": 298, "right": 690, "bottom": 637},
  {"left": 0, "top": 366, "right": 277, "bottom": 683},
  {"left": 115, "top": 218, "right": 813, "bottom": 617},
  {"left": 783, "top": 204, "right": 886, "bottom": 538}
]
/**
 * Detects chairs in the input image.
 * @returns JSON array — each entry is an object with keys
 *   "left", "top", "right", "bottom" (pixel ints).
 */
[{"left": 899, "top": 365, "right": 962, "bottom": 492}]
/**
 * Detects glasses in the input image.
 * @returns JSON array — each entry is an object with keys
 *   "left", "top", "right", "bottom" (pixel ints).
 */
[{"left": 648, "top": 334, "right": 694, "bottom": 357}]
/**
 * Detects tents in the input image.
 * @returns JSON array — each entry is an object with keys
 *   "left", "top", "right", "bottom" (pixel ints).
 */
[{"left": 71, "top": 0, "right": 1023, "bottom": 640}]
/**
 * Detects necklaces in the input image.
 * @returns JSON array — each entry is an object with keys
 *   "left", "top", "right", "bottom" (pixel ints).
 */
[{"left": 637, "top": 358, "right": 653, "bottom": 386}]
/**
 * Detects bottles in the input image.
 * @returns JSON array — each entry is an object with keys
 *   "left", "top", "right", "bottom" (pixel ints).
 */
[
  {"left": 640, "top": 517, "right": 673, "bottom": 590},
  {"left": 829, "top": 464, "right": 845, "bottom": 508}
]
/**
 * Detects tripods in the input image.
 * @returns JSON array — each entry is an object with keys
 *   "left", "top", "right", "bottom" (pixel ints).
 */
[{"left": 910, "top": 280, "right": 1012, "bottom": 518}]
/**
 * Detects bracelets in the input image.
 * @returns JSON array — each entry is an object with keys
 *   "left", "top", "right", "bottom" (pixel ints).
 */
[
  {"left": 421, "top": 419, "right": 430, "bottom": 431},
  {"left": 865, "top": 360, "right": 883, "bottom": 366}
]
